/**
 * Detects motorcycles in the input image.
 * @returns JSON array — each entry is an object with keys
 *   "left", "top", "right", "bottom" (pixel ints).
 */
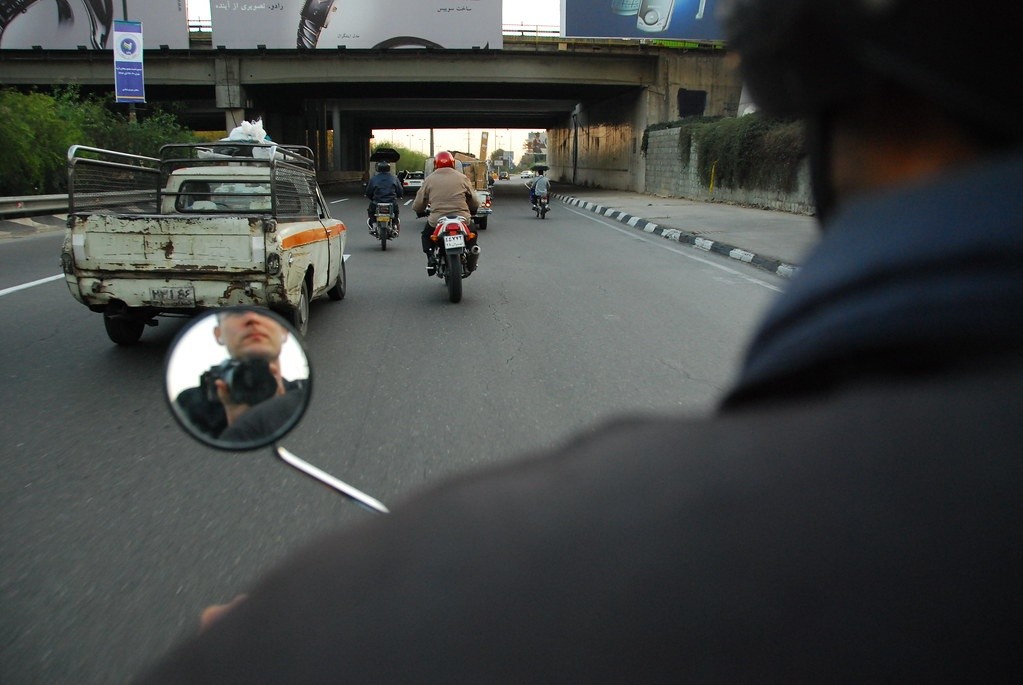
[
  {"left": 415, "top": 204, "right": 481, "bottom": 303},
  {"left": 528, "top": 188, "right": 552, "bottom": 220},
  {"left": 362, "top": 181, "right": 402, "bottom": 251}
]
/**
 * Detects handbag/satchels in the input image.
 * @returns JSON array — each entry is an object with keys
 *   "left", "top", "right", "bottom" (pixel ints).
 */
[{"left": 531, "top": 181, "right": 536, "bottom": 193}]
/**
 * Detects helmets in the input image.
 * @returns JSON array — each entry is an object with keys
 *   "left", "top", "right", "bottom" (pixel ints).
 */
[
  {"left": 434, "top": 151, "right": 455, "bottom": 169},
  {"left": 376, "top": 158, "right": 391, "bottom": 172}
]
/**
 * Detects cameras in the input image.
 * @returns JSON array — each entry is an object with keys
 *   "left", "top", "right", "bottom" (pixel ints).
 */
[{"left": 200, "top": 358, "right": 278, "bottom": 406}]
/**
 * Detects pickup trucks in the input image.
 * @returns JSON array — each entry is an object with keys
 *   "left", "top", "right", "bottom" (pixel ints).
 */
[{"left": 60, "top": 117, "right": 351, "bottom": 343}]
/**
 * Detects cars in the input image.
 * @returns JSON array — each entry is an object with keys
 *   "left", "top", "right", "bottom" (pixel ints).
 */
[
  {"left": 520, "top": 170, "right": 534, "bottom": 179},
  {"left": 499, "top": 171, "right": 511, "bottom": 181},
  {"left": 402, "top": 170, "right": 424, "bottom": 195}
]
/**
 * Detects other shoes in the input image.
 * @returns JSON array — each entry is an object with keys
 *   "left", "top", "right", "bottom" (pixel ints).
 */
[
  {"left": 532, "top": 206, "right": 536, "bottom": 210},
  {"left": 427, "top": 255, "right": 438, "bottom": 276}
]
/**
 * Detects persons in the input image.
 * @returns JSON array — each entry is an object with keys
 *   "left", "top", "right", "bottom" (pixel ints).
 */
[
  {"left": 413, "top": 151, "right": 482, "bottom": 275},
  {"left": 128, "top": 0, "right": 1022, "bottom": 685},
  {"left": 171, "top": 310, "right": 308, "bottom": 444},
  {"left": 366, "top": 160, "right": 403, "bottom": 233},
  {"left": 530, "top": 170, "right": 551, "bottom": 211}
]
